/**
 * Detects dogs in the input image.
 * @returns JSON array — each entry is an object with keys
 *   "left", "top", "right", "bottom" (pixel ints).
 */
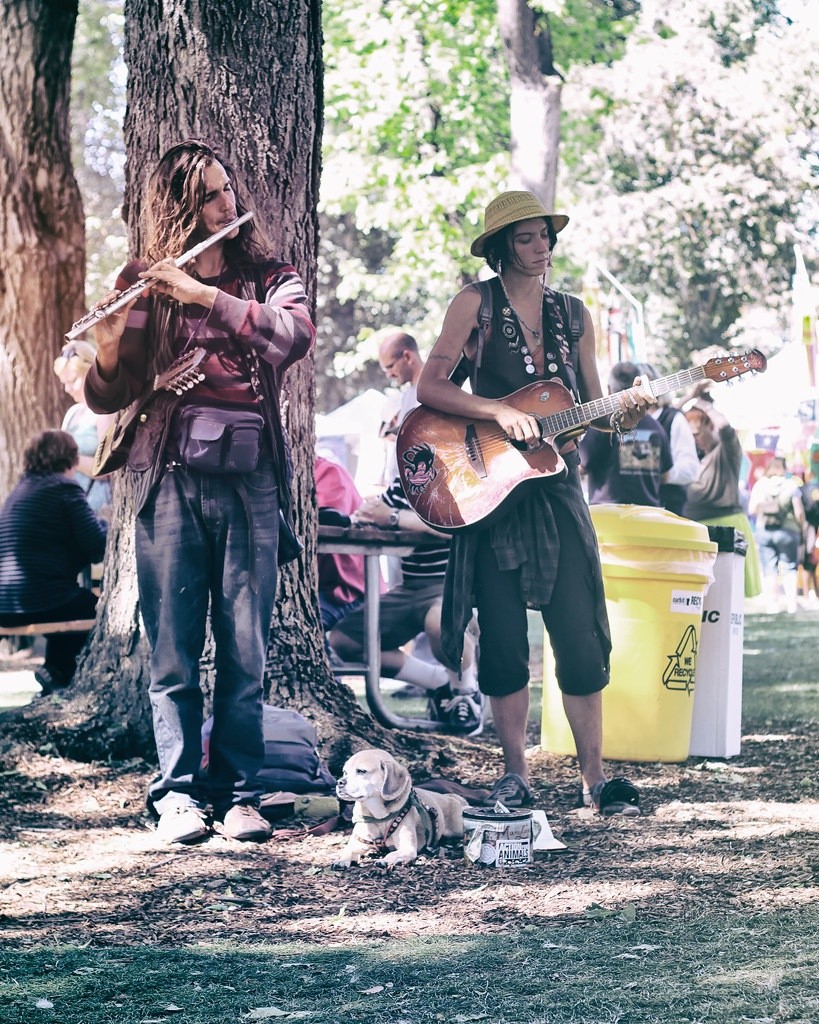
[{"left": 330, "top": 749, "right": 474, "bottom": 871}]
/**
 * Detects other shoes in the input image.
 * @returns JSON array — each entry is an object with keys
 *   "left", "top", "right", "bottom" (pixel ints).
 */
[
  {"left": 222, "top": 804, "right": 273, "bottom": 840},
  {"left": 580, "top": 777, "right": 641, "bottom": 817},
  {"left": 35, "top": 666, "right": 59, "bottom": 694},
  {"left": 483, "top": 772, "right": 531, "bottom": 807},
  {"left": 156, "top": 804, "right": 209, "bottom": 846}
]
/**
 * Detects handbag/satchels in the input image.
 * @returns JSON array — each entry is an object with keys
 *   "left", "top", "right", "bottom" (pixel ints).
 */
[{"left": 171, "top": 401, "right": 265, "bottom": 480}]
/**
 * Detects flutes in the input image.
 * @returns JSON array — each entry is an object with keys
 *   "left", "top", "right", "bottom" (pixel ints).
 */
[{"left": 63, "top": 212, "right": 256, "bottom": 343}]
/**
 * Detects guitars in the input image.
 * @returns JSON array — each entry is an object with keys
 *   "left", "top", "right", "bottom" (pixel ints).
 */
[
  {"left": 395, "top": 347, "right": 767, "bottom": 535},
  {"left": 91, "top": 345, "right": 210, "bottom": 477}
]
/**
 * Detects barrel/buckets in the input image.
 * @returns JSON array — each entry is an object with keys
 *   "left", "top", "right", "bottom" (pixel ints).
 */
[{"left": 464, "top": 805, "right": 534, "bottom": 870}]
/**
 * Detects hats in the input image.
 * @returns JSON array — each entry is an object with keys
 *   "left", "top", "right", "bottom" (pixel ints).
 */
[{"left": 470, "top": 190, "right": 570, "bottom": 258}]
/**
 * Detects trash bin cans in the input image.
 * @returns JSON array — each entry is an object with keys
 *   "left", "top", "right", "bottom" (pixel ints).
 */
[
  {"left": 690, "top": 526, "right": 748, "bottom": 759},
  {"left": 540, "top": 502, "right": 719, "bottom": 763}
]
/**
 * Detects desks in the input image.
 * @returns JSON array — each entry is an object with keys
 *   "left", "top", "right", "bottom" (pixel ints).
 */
[{"left": 315, "top": 525, "right": 450, "bottom": 731}]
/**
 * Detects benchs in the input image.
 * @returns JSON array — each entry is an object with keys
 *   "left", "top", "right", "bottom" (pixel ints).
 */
[{"left": 0, "top": 619, "right": 97, "bottom": 635}]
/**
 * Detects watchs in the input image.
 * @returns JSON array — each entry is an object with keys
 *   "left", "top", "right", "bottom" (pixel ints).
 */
[{"left": 390, "top": 509, "right": 401, "bottom": 529}]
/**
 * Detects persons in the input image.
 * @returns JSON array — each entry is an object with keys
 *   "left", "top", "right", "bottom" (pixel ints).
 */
[
  {"left": 54, "top": 340, "right": 113, "bottom": 514},
  {"left": 314, "top": 456, "right": 387, "bottom": 631},
  {"left": 675, "top": 380, "right": 763, "bottom": 598},
  {"left": 85, "top": 141, "right": 317, "bottom": 844},
  {"left": 0, "top": 429, "right": 106, "bottom": 691},
  {"left": 748, "top": 456, "right": 808, "bottom": 616},
  {"left": 329, "top": 474, "right": 484, "bottom": 736},
  {"left": 416, "top": 191, "right": 658, "bottom": 816},
  {"left": 579, "top": 362, "right": 674, "bottom": 508},
  {"left": 378, "top": 332, "right": 426, "bottom": 488},
  {"left": 635, "top": 363, "right": 700, "bottom": 518}
]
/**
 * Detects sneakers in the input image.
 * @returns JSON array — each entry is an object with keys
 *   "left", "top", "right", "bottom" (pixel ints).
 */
[
  {"left": 425, "top": 682, "right": 458, "bottom": 725},
  {"left": 439, "top": 689, "right": 489, "bottom": 738}
]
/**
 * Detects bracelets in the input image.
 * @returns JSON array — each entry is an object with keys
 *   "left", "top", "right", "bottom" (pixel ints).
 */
[{"left": 609, "top": 411, "right": 638, "bottom": 435}]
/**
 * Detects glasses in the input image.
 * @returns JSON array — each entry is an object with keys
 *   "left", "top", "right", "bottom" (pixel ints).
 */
[{"left": 384, "top": 357, "right": 400, "bottom": 370}]
